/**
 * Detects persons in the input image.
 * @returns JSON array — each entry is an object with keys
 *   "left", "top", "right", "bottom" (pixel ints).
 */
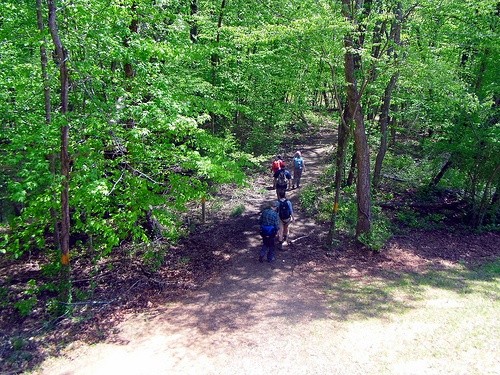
[
  {"left": 273, "top": 162, "right": 292, "bottom": 202},
  {"left": 291, "top": 150, "right": 306, "bottom": 190},
  {"left": 258, "top": 201, "right": 280, "bottom": 264},
  {"left": 275, "top": 191, "right": 296, "bottom": 251},
  {"left": 272, "top": 154, "right": 285, "bottom": 174}
]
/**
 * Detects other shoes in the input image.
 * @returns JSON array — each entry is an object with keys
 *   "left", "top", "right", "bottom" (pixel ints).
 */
[
  {"left": 259, "top": 255, "right": 263, "bottom": 263},
  {"left": 268, "top": 258, "right": 275, "bottom": 263}
]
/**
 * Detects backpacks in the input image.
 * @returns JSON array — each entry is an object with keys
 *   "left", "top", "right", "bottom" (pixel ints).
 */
[
  {"left": 293, "top": 157, "right": 303, "bottom": 170},
  {"left": 277, "top": 171, "right": 288, "bottom": 187},
  {"left": 277, "top": 199, "right": 290, "bottom": 220}
]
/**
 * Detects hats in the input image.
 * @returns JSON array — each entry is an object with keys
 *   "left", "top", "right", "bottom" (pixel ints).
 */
[{"left": 270, "top": 201, "right": 280, "bottom": 207}]
